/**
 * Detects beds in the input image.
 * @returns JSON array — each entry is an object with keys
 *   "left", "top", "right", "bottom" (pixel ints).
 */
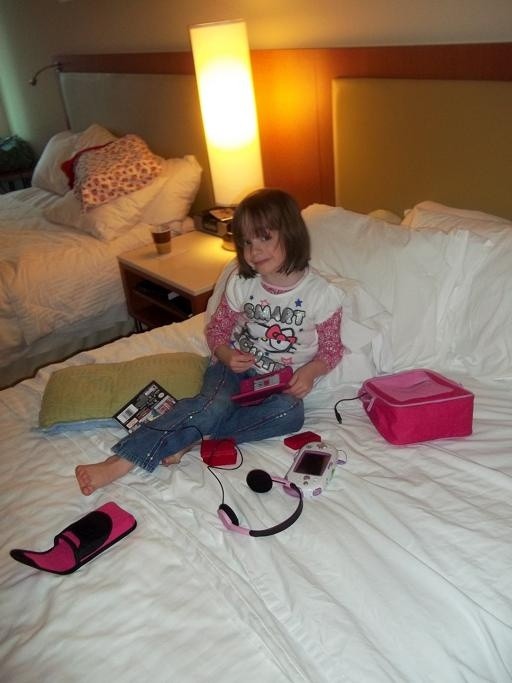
[{"left": 0, "top": 211, "right": 512, "bottom": 683}]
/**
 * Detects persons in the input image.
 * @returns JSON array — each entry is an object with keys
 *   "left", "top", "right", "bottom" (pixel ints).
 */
[{"left": 75, "top": 188, "right": 344, "bottom": 496}]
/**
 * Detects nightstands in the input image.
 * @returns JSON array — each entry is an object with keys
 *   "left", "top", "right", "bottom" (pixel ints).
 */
[{"left": 116, "top": 230, "right": 240, "bottom": 330}]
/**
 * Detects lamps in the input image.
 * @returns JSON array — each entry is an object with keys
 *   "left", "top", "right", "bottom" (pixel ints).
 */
[{"left": 186, "top": 16, "right": 267, "bottom": 252}]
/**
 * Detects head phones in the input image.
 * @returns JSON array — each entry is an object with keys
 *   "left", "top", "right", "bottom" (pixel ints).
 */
[{"left": 217, "top": 469, "right": 303, "bottom": 538}]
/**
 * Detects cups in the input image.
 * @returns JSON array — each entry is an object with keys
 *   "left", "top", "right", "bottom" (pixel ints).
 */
[{"left": 149, "top": 225, "right": 172, "bottom": 256}]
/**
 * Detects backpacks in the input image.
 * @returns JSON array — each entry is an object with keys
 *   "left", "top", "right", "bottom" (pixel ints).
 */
[{"left": 359, "top": 368, "right": 474, "bottom": 446}]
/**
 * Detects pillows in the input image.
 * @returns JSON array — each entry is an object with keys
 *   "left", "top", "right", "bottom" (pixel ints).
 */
[
  {"left": 39, "top": 353, "right": 209, "bottom": 433},
  {"left": 404, "top": 202, "right": 512, "bottom": 379},
  {"left": 298, "top": 201, "right": 485, "bottom": 377},
  {"left": 73, "top": 133, "right": 157, "bottom": 209},
  {"left": 151, "top": 154, "right": 201, "bottom": 225},
  {"left": 0, "top": 127, "right": 196, "bottom": 389},
  {"left": 33, "top": 132, "right": 90, "bottom": 194},
  {"left": 44, "top": 175, "right": 164, "bottom": 241}
]
